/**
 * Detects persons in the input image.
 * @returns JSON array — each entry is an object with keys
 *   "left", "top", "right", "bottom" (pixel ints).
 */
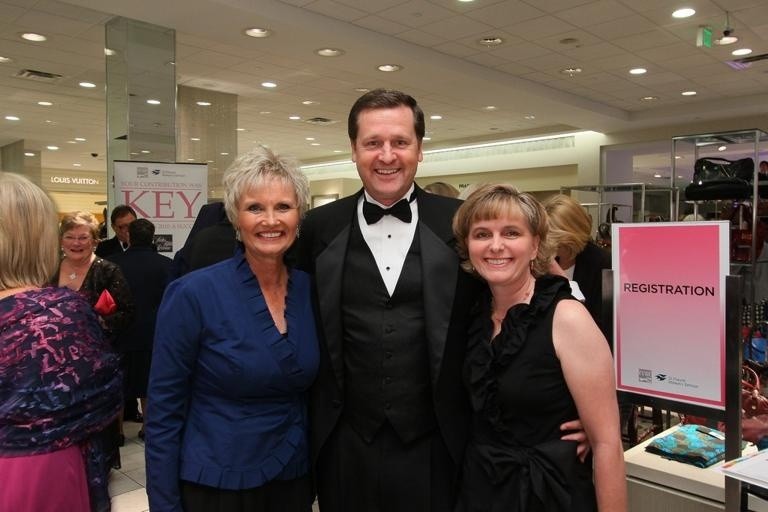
[
  {"left": 298, "top": 84, "right": 590, "bottom": 512},
  {"left": 757, "top": 160, "right": 768, "bottom": 198},
  {"left": 105, "top": 216, "right": 173, "bottom": 442},
  {"left": 541, "top": 192, "right": 602, "bottom": 341},
  {"left": 0, "top": 172, "right": 128, "bottom": 512},
  {"left": 50, "top": 210, "right": 132, "bottom": 451},
  {"left": 448, "top": 182, "right": 630, "bottom": 512},
  {"left": 146, "top": 143, "right": 325, "bottom": 512},
  {"left": 95, "top": 203, "right": 140, "bottom": 422}
]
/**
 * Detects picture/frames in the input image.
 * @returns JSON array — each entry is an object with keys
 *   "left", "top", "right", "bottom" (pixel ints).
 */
[{"left": 311, "top": 192, "right": 340, "bottom": 209}]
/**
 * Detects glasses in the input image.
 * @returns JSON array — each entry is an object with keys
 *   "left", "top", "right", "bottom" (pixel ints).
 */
[
  {"left": 113, "top": 223, "right": 129, "bottom": 230},
  {"left": 64, "top": 234, "right": 92, "bottom": 242}
]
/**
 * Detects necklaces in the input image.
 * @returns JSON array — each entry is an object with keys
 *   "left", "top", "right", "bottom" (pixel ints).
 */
[
  {"left": 492, "top": 275, "right": 532, "bottom": 322},
  {"left": 64, "top": 257, "right": 92, "bottom": 280}
]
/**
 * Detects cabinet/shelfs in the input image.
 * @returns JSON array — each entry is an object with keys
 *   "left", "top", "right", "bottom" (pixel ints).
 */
[
  {"left": 669, "top": 127, "right": 767, "bottom": 327},
  {"left": 557, "top": 182, "right": 679, "bottom": 252}
]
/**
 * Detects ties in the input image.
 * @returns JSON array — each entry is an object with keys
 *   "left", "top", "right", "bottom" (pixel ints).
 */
[{"left": 356, "top": 198, "right": 414, "bottom": 227}]
